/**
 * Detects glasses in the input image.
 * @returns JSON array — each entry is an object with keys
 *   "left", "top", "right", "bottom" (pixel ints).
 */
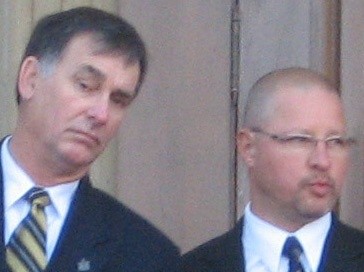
[{"left": 249, "top": 128, "right": 358, "bottom": 160}]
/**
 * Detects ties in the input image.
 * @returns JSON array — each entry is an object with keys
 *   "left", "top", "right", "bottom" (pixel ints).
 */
[
  {"left": 6, "top": 187, "right": 50, "bottom": 272},
  {"left": 282, "top": 236, "right": 305, "bottom": 272}
]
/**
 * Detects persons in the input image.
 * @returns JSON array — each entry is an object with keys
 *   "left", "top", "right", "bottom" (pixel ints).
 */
[
  {"left": 176, "top": 66, "right": 364, "bottom": 272},
  {"left": 0, "top": 6, "right": 182, "bottom": 272}
]
